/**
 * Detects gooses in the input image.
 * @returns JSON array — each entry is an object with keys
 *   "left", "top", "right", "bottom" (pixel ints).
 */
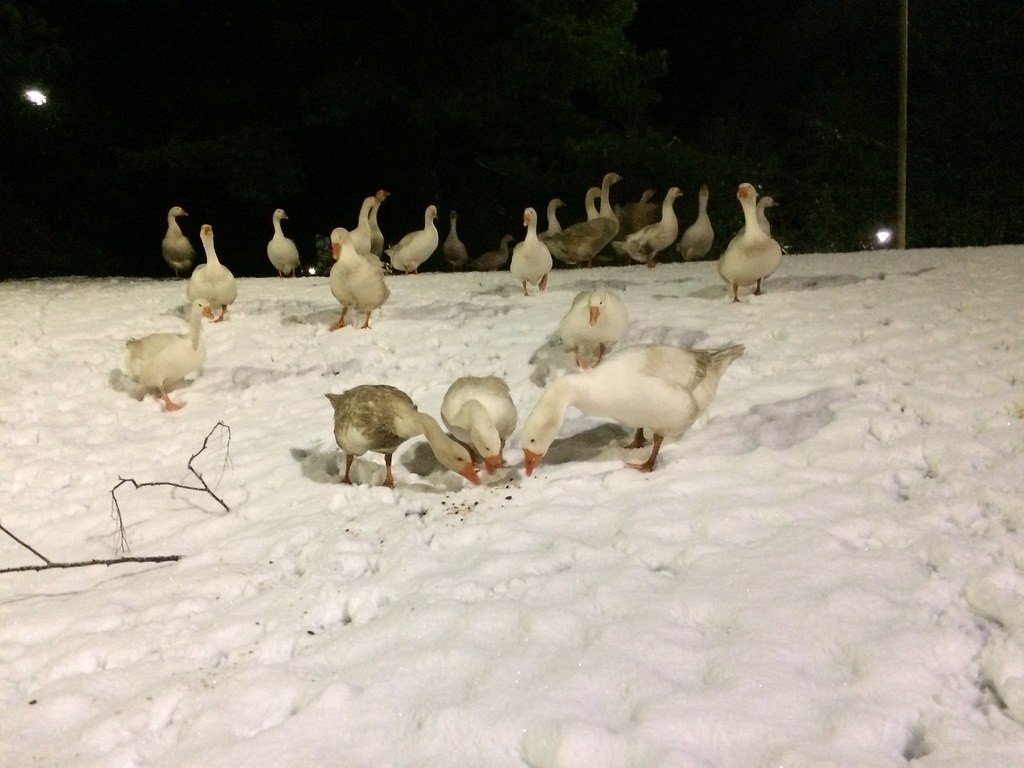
[{"left": 124, "top": 172, "right": 781, "bottom": 486}]
